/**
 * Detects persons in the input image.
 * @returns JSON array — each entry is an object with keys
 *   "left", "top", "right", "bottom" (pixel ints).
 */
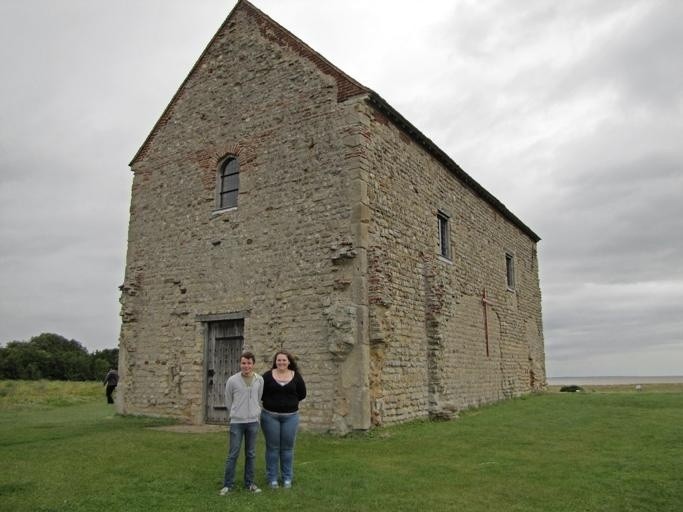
[
  {"left": 219, "top": 351, "right": 265, "bottom": 495},
  {"left": 103, "top": 367, "right": 119, "bottom": 404},
  {"left": 260, "top": 350, "right": 306, "bottom": 489}
]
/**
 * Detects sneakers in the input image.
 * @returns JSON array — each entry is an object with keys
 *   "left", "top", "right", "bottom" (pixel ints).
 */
[
  {"left": 218, "top": 487, "right": 230, "bottom": 495},
  {"left": 269, "top": 481, "right": 292, "bottom": 489},
  {"left": 248, "top": 484, "right": 261, "bottom": 492}
]
